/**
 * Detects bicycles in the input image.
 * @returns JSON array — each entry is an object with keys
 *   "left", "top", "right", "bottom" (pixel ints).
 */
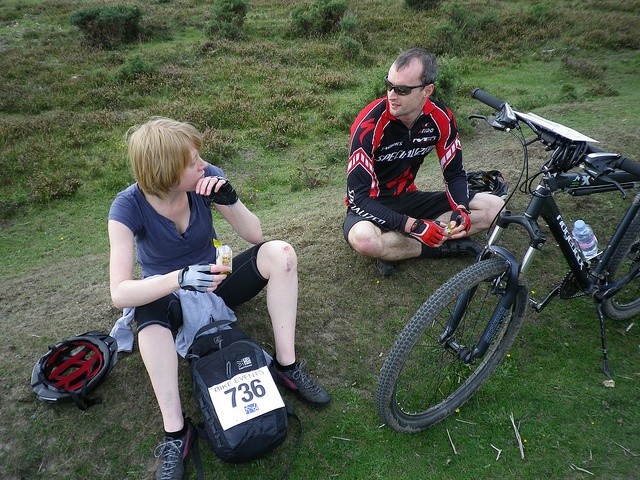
[{"left": 376, "top": 86, "right": 640, "bottom": 435}]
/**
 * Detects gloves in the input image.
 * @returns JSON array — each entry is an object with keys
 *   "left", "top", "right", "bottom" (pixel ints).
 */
[
  {"left": 397, "top": 214, "right": 445, "bottom": 247},
  {"left": 200, "top": 175, "right": 238, "bottom": 205},
  {"left": 178, "top": 261, "right": 213, "bottom": 293},
  {"left": 449, "top": 202, "right": 471, "bottom": 237}
]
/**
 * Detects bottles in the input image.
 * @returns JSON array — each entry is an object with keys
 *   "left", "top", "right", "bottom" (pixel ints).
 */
[{"left": 569, "top": 217, "right": 601, "bottom": 260}]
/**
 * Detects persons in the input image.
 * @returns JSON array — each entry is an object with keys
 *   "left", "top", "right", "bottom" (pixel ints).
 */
[
  {"left": 108, "top": 116, "right": 331, "bottom": 480},
  {"left": 344, "top": 47, "right": 507, "bottom": 262}
]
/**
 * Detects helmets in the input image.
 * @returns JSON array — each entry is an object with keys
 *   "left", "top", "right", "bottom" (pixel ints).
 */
[
  {"left": 30, "top": 330, "right": 118, "bottom": 411},
  {"left": 466, "top": 170, "right": 511, "bottom": 201}
]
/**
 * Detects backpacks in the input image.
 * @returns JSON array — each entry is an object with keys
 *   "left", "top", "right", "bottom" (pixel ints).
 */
[{"left": 186, "top": 314, "right": 303, "bottom": 480}]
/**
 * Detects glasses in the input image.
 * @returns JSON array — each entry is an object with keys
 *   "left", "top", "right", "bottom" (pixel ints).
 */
[{"left": 384, "top": 75, "right": 435, "bottom": 96}]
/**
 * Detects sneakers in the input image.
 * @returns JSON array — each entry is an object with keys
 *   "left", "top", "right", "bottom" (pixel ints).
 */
[
  {"left": 434, "top": 237, "right": 484, "bottom": 261},
  {"left": 153, "top": 412, "right": 198, "bottom": 479},
  {"left": 377, "top": 258, "right": 393, "bottom": 278},
  {"left": 268, "top": 353, "right": 331, "bottom": 406}
]
